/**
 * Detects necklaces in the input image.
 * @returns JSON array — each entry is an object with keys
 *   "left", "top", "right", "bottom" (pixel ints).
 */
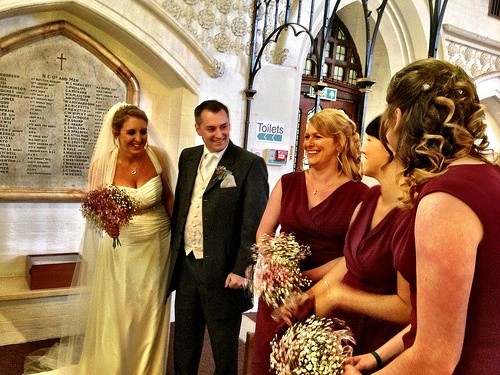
[
  {"left": 130, "top": 166, "right": 138, "bottom": 174},
  {"left": 313, "top": 189, "right": 318, "bottom": 195}
]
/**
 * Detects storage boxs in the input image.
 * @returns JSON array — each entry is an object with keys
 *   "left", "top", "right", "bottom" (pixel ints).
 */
[{"left": 23, "top": 252, "right": 86, "bottom": 290}]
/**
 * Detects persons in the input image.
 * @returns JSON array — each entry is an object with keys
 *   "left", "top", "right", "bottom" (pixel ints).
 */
[
  {"left": 23, "top": 103, "right": 178, "bottom": 375},
  {"left": 280, "top": 113, "right": 416, "bottom": 375},
  {"left": 341, "top": 58, "right": 500, "bottom": 375},
  {"left": 164, "top": 100, "right": 270, "bottom": 375},
  {"left": 249, "top": 108, "right": 371, "bottom": 375}
]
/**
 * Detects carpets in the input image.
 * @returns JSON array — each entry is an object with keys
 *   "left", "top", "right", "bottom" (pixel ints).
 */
[{"left": 0, "top": 322, "right": 246, "bottom": 375}]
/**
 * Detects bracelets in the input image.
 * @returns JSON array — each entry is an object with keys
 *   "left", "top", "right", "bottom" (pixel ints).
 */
[{"left": 371, "top": 352, "right": 383, "bottom": 367}]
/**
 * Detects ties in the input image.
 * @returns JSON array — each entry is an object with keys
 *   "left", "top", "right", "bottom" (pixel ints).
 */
[{"left": 200, "top": 153, "right": 215, "bottom": 181}]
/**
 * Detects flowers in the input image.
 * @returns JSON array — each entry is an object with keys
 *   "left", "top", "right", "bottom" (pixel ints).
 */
[
  {"left": 80, "top": 184, "right": 140, "bottom": 249},
  {"left": 269, "top": 315, "right": 357, "bottom": 375},
  {"left": 244, "top": 230, "right": 312, "bottom": 311},
  {"left": 212, "top": 166, "right": 232, "bottom": 182}
]
